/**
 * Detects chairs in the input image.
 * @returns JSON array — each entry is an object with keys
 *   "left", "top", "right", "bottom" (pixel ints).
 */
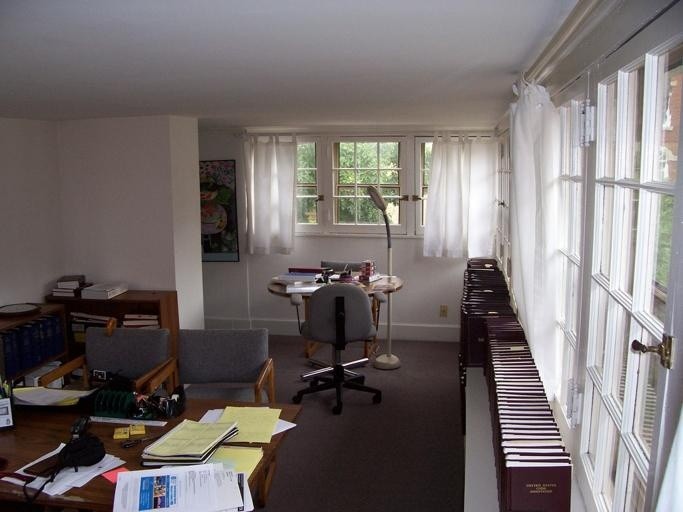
[
  {"left": 290, "top": 260, "right": 385, "bottom": 416},
  {"left": 37, "top": 326, "right": 276, "bottom": 402}
]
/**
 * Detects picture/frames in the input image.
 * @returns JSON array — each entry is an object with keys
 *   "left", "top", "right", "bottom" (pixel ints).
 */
[{"left": 199, "top": 159, "right": 240, "bottom": 264}]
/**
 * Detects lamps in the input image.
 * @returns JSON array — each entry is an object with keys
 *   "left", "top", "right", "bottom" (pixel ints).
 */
[{"left": 367, "top": 185, "right": 401, "bottom": 370}]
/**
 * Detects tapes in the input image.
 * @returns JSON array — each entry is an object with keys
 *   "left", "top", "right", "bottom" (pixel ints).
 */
[{"left": 171, "top": 394, "right": 180, "bottom": 403}]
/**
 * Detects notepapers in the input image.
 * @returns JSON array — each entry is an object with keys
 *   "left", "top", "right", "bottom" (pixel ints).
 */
[{"left": 113, "top": 424, "right": 146, "bottom": 440}]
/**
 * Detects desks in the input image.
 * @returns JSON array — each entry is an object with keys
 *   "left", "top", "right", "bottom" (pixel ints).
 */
[
  {"left": 1, "top": 402, "right": 302, "bottom": 511},
  {"left": 267, "top": 274, "right": 404, "bottom": 362}
]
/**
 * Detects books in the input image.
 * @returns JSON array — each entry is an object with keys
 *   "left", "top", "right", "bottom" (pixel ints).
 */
[
  {"left": 140, "top": 419, "right": 240, "bottom": 467},
  {"left": 52, "top": 274, "right": 128, "bottom": 300},
  {"left": 461, "top": 258, "right": 573, "bottom": 512},
  {"left": 268, "top": 267, "right": 332, "bottom": 295},
  {"left": 69, "top": 310, "right": 160, "bottom": 330},
  {"left": 2, "top": 312, "right": 61, "bottom": 374}
]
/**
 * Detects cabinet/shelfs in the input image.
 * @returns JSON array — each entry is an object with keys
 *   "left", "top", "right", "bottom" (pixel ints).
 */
[{"left": 0, "top": 288, "right": 178, "bottom": 383}]
